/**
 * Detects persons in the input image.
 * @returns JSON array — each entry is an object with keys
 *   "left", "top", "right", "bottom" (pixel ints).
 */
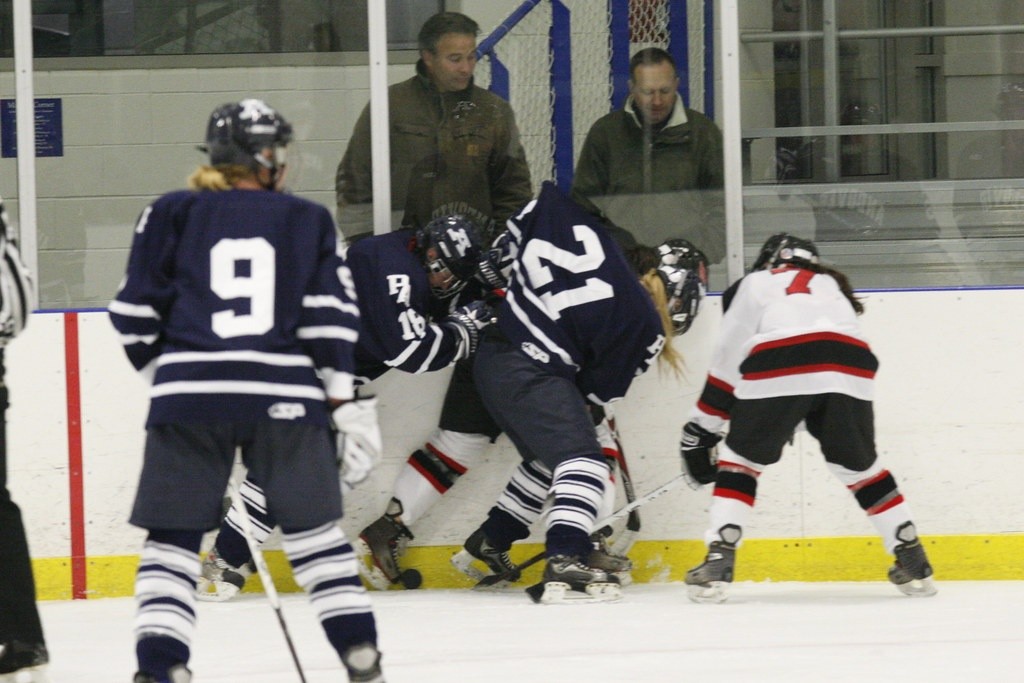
[
  {"left": 353, "top": 185, "right": 710, "bottom": 603},
  {"left": 569, "top": 47, "right": 727, "bottom": 265},
  {"left": 679, "top": 233, "right": 938, "bottom": 603},
  {"left": 194, "top": 215, "right": 495, "bottom": 602},
  {"left": 0, "top": 203, "right": 50, "bottom": 683},
  {"left": 106, "top": 99, "right": 385, "bottom": 683},
  {"left": 335, "top": 12, "right": 534, "bottom": 232},
  {"left": 954, "top": 82, "right": 1024, "bottom": 285},
  {"left": 774, "top": 98, "right": 962, "bottom": 287}
]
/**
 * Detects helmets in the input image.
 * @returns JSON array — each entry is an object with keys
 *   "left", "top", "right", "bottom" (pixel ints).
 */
[
  {"left": 751, "top": 233, "right": 819, "bottom": 271},
  {"left": 647, "top": 237, "right": 705, "bottom": 335},
  {"left": 208, "top": 100, "right": 291, "bottom": 190},
  {"left": 415, "top": 214, "right": 482, "bottom": 297}
]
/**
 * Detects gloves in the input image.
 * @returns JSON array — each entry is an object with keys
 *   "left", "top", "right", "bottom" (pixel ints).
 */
[
  {"left": 455, "top": 299, "right": 496, "bottom": 331},
  {"left": 328, "top": 394, "right": 382, "bottom": 485},
  {"left": 680, "top": 420, "right": 722, "bottom": 485}
]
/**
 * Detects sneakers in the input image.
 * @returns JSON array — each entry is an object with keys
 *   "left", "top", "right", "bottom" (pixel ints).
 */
[
  {"left": 195, "top": 546, "right": 241, "bottom": 603},
  {"left": 351, "top": 516, "right": 413, "bottom": 593},
  {"left": 571, "top": 534, "right": 632, "bottom": 588},
  {"left": 887, "top": 527, "right": 937, "bottom": 596},
  {"left": 684, "top": 525, "right": 741, "bottom": 604},
  {"left": 537, "top": 555, "right": 622, "bottom": 604},
  {"left": 451, "top": 526, "right": 519, "bottom": 588},
  {"left": 345, "top": 646, "right": 386, "bottom": 683},
  {"left": 0, "top": 636, "right": 52, "bottom": 682}
]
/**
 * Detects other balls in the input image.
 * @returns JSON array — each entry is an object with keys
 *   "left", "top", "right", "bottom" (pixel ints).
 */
[{"left": 401, "top": 569, "right": 422, "bottom": 589}]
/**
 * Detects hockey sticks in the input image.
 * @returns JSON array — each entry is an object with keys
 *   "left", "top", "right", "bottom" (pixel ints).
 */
[
  {"left": 472, "top": 474, "right": 687, "bottom": 591},
  {"left": 603, "top": 402, "right": 641, "bottom": 556},
  {"left": 226, "top": 476, "right": 306, "bottom": 683}
]
